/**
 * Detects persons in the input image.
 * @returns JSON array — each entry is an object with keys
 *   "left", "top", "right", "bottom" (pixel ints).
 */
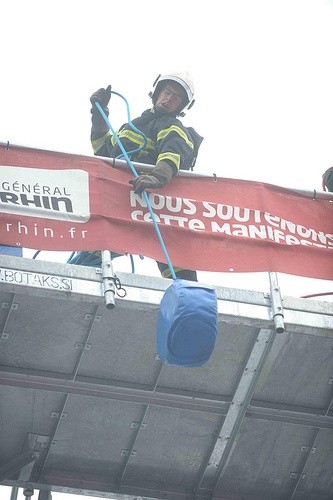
[
  {"left": 322, "top": 166, "right": 333, "bottom": 193},
  {"left": 66, "top": 74, "right": 198, "bottom": 283}
]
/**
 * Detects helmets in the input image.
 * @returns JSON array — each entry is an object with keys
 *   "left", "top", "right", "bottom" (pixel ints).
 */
[{"left": 148, "top": 72, "right": 196, "bottom": 117}]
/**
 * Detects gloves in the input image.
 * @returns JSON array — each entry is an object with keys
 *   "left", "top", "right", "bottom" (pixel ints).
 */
[
  {"left": 129, "top": 161, "right": 174, "bottom": 194},
  {"left": 90, "top": 85, "right": 112, "bottom": 132}
]
